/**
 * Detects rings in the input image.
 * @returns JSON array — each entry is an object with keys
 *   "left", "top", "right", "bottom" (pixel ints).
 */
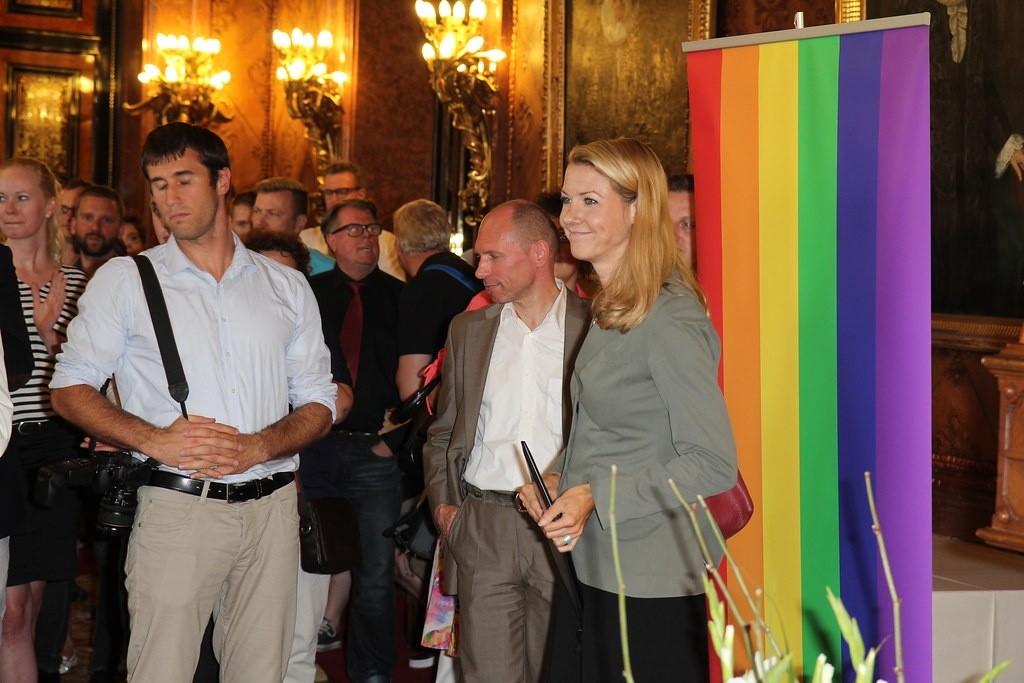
[{"left": 562, "top": 534, "right": 572, "bottom": 544}]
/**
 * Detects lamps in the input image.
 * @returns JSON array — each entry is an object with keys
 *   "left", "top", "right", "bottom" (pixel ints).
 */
[
  {"left": 120, "top": 33, "right": 233, "bottom": 131},
  {"left": 274, "top": 29, "right": 347, "bottom": 221},
  {"left": 414, "top": 0, "right": 506, "bottom": 226}
]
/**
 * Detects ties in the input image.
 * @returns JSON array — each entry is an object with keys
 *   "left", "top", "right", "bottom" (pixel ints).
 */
[{"left": 340, "top": 277, "right": 373, "bottom": 403}]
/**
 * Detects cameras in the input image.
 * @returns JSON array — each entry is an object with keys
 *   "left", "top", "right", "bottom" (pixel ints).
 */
[{"left": 38, "top": 450, "right": 139, "bottom": 535}]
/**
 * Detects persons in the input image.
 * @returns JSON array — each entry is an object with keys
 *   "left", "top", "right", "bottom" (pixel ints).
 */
[
  {"left": 0, "top": 159, "right": 696, "bottom": 683},
  {"left": 48, "top": 123, "right": 338, "bottom": 683},
  {"left": 519, "top": 138, "right": 738, "bottom": 683}
]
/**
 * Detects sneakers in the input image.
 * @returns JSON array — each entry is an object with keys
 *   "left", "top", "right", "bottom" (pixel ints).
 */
[{"left": 317, "top": 619, "right": 342, "bottom": 651}]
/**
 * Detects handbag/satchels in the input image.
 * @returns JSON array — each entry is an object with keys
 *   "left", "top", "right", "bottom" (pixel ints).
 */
[
  {"left": 297, "top": 499, "right": 362, "bottom": 575},
  {"left": 702, "top": 467, "right": 753, "bottom": 540}
]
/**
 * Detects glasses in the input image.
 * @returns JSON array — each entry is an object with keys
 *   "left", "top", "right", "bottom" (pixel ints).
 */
[
  {"left": 324, "top": 187, "right": 361, "bottom": 195},
  {"left": 328, "top": 224, "right": 383, "bottom": 237},
  {"left": 62, "top": 205, "right": 75, "bottom": 214}
]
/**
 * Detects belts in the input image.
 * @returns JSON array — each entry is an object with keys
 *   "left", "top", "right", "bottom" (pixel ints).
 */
[
  {"left": 142, "top": 467, "right": 296, "bottom": 504},
  {"left": 460, "top": 475, "right": 529, "bottom": 513},
  {"left": 11, "top": 419, "right": 71, "bottom": 435}
]
